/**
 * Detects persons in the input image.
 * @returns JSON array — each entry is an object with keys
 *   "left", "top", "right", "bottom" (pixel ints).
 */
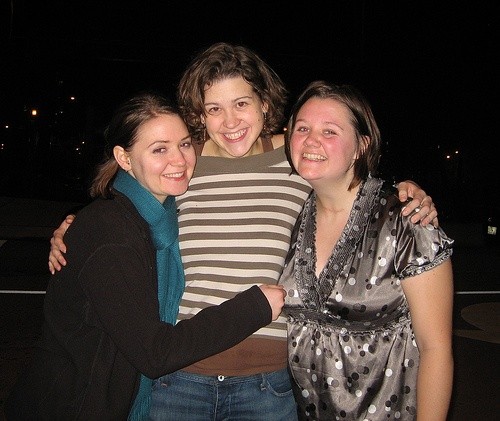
[
  {"left": 4, "top": 89, "right": 287, "bottom": 421},
  {"left": 278, "top": 80, "right": 455, "bottom": 421},
  {"left": 47, "top": 43, "right": 439, "bottom": 420}
]
